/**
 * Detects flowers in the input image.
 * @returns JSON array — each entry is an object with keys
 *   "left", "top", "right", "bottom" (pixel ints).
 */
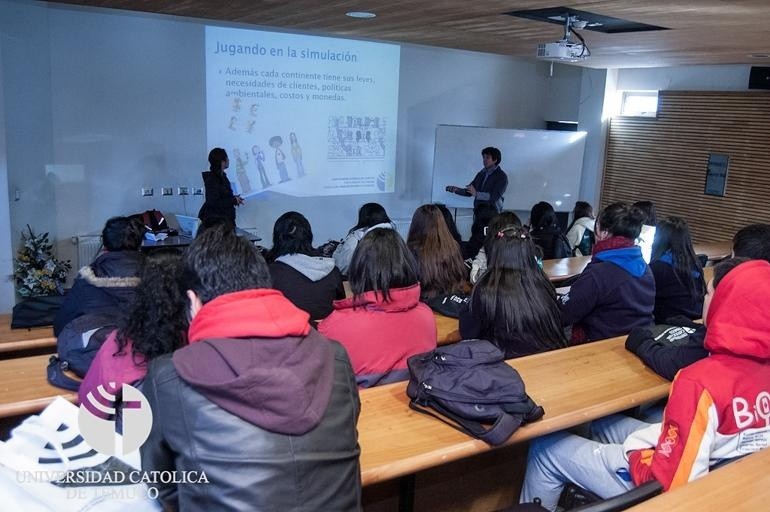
[{"left": 11, "top": 223, "right": 74, "bottom": 295}]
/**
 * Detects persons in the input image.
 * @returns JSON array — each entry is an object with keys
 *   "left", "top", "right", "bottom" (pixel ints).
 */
[
  {"left": 268, "top": 136, "right": 292, "bottom": 185},
  {"left": 314, "top": 199, "right": 770, "bottom": 391},
  {"left": 220, "top": 158, "right": 242, "bottom": 198},
  {"left": 516, "top": 257, "right": 770, "bottom": 512},
  {"left": 251, "top": 146, "right": 274, "bottom": 188},
  {"left": 76, "top": 250, "right": 197, "bottom": 451},
  {"left": 261, "top": 210, "right": 346, "bottom": 329},
  {"left": 444, "top": 146, "right": 509, "bottom": 260},
  {"left": 122, "top": 213, "right": 155, "bottom": 249},
  {"left": 196, "top": 147, "right": 246, "bottom": 236},
  {"left": 134, "top": 221, "right": 366, "bottom": 512},
  {"left": 51, "top": 215, "right": 156, "bottom": 341},
  {"left": 230, "top": 148, "right": 255, "bottom": 194},
  {"left": 288, "top": 132, "right": 307, "bottom": 178}
]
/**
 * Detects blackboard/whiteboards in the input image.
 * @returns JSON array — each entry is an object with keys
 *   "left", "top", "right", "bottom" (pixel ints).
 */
[{"left": 430, "top": 124, "right": 588, "bottom": 212}]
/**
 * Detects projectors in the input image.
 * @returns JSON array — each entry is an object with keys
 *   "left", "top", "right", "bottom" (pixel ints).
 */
[{"left": 535, "top": 42, "right": 589, "bottom": 62}]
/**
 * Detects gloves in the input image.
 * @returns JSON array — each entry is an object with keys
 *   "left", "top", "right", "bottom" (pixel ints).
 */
[
  {"left": 665, "top": 315, "right": 698, "bottom": 330},
  {"left": 624, "top": 328, "right": 653, "bottom": 353}
]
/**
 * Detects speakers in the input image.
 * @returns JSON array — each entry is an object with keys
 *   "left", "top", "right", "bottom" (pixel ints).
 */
[{"left": 747, "top": 66, "right": 770, "bottom": 91}]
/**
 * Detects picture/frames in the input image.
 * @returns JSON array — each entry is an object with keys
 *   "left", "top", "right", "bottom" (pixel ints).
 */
[{"left": 702, "top": 151, "right": 730, "bottom": 196}]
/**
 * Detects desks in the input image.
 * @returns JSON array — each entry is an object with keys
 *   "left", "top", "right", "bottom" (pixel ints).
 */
[{"left": 139, "top": 227, "right": 263, "bottom": 250}]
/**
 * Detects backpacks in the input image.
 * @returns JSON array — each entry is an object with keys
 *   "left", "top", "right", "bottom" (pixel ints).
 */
[
  {"left": 47, "top": 314, "right": 120, "bottom": 393},
  {"left": 407, "top": 339, "right": 544, "bottom": 446},
  {"left": 141, "top": 210, "right": 169, "bottom": 240},
  {"left": 11, "top": 297, "right": 65, "bottom": 328}
]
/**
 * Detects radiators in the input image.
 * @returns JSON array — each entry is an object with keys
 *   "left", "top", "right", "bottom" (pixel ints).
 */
[{"left": 70, "top": 234, "right": 102, "bottom": 269}]
